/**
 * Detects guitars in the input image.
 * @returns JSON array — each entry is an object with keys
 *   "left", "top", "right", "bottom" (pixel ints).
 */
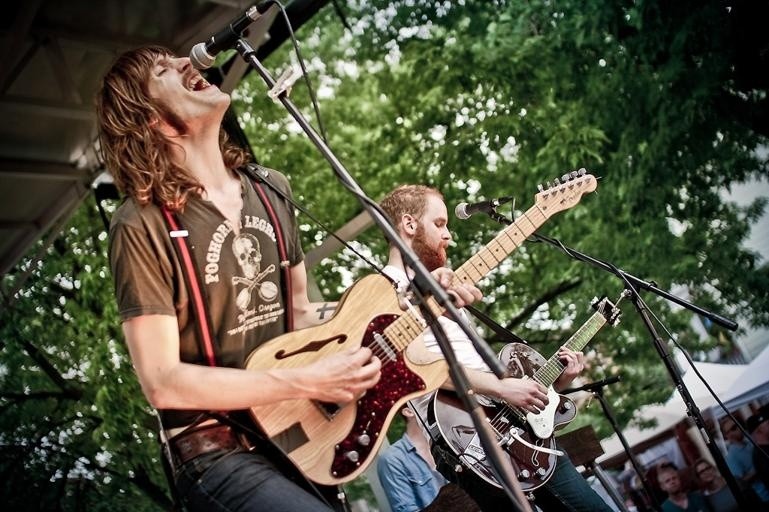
[
  {"left": 243, "top": 168, "right": 598, "bottom": 485},
  {"left": 427, "top": 296, "right": 623, "bottom": 498}
]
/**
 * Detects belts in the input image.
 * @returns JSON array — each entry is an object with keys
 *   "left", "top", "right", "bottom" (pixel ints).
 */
[{"left": 164, "top": 424, "right": 238, "bottom": 468}]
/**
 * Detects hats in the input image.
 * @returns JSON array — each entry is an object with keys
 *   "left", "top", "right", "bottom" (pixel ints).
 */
[{"left": 744, "top": 415, "right": 767, "bottom": 435}]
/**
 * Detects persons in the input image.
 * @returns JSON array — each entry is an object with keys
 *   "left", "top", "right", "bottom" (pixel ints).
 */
[
  {"left": 377, "top": 182, "right": 614, "bottom": 512},
  {"left": 379, "top": 400, "right": 481, "bottom": 512},
  {"left": 92, "top": 43, "right": 485, "bottom": 512},
  {"left": 606, "top": 401, "right": 769, "bottom": 512}
]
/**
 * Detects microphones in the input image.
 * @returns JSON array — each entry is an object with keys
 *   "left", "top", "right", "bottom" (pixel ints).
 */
[
  {"left": 454, "top": 195, "right": 513, "bottom": 220},
  {"left": 189, "top": 0, "right": 276, "bottom": 70}
]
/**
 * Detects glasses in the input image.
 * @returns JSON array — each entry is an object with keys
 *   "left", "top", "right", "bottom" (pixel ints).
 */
[
  {"left": 697, "top": 466, "right": 711, "bottom": 475},
  {"left": 725, "top": 424, "right": 737, "bottom": 434}
]
